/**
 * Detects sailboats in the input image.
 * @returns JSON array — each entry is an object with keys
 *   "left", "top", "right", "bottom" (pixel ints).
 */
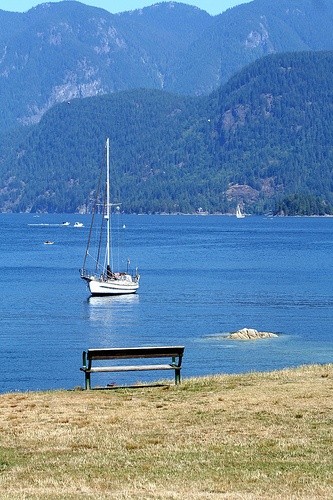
[
  {"left": 236, "top": 205, "right": 245, "bottom": 218},
  {"left": 79, "top": 137, "right": 142, "bottom": 297}
]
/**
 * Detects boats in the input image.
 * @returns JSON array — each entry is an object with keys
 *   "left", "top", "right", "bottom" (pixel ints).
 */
[
  {"left": 74, "top": 221, "right": 84, "bottom": 227},
  {"left": 43, "top": 240, "right": 54, "bottom": 245},
  {"left": 62, "top": 221, "right": 70, "bottom": 226}
]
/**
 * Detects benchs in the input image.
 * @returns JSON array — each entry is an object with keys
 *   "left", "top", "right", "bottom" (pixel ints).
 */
[{"left": 79, "top": 347, "right": 182, "bottom": 391}]
